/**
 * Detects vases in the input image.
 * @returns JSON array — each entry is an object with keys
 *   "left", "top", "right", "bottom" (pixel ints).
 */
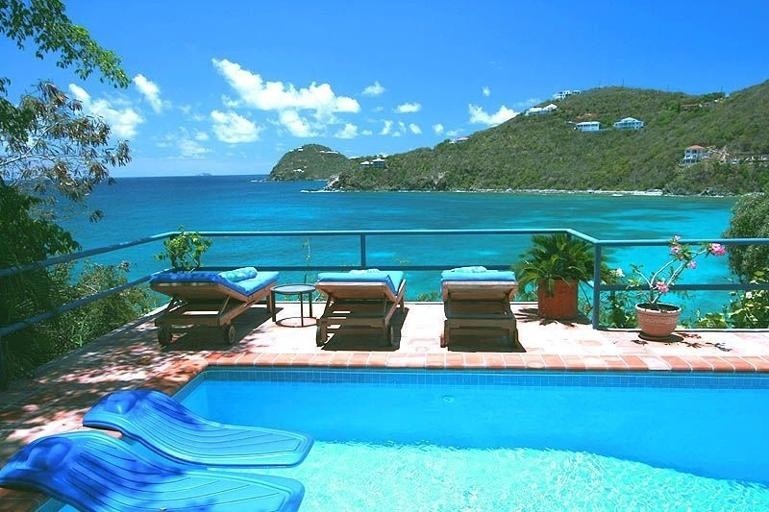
[{"left": 634, "top": 303, "right": 682, "bottom": 341}]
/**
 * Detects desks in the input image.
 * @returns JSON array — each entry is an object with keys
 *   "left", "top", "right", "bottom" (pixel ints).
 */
[{"left": 269, "top": 283, "right": 317, "bottom": 327}]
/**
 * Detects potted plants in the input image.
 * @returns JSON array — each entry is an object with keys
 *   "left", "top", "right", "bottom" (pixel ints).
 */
[{"left": 512, "top": 231, "right": 618, "bottom": 321}]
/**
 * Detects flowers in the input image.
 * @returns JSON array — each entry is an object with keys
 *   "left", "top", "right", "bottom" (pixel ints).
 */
[{"left": 626, "top": 234, "right": 726, "bottom": 310}]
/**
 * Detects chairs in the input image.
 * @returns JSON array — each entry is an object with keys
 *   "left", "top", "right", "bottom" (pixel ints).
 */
[
  {"left": 441, "top": 267, "right": 517, "bottom": 348},
  {"left": 149, "top": 270, "right": 280, "bottom": 344},
  {"left": 313, "top": 270, "right": 406, "bottom": 349}
]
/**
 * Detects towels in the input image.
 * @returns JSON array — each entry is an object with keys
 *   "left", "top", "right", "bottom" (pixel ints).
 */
[{"left": 219, "top": 266, "right": 257, "bottom": 282}]
